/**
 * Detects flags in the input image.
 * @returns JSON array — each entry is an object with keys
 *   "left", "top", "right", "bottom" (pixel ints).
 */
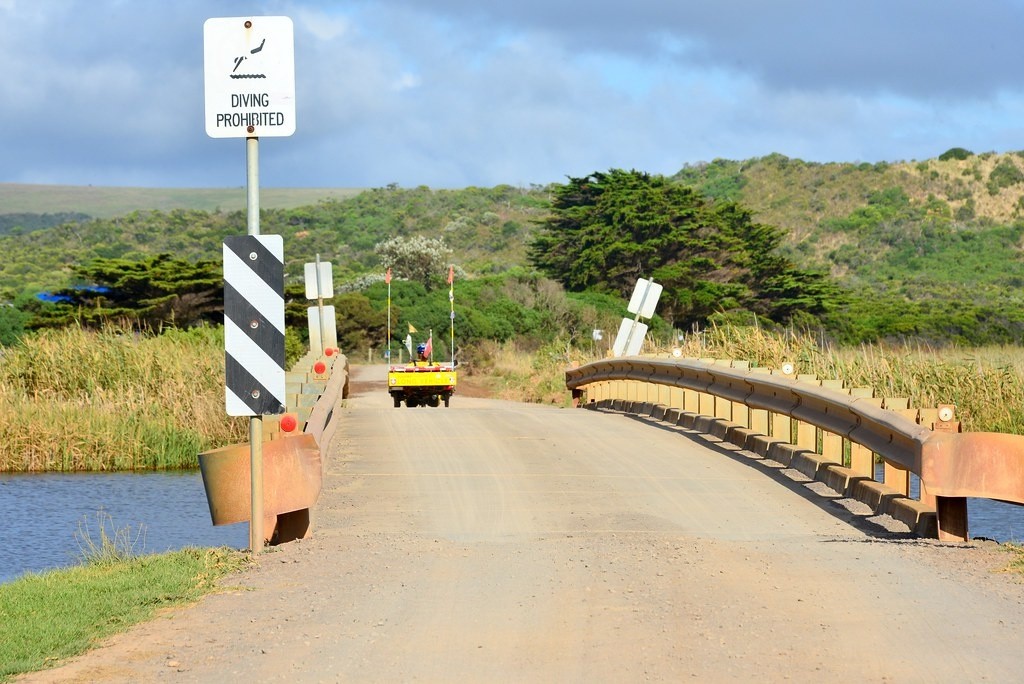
[
  {"left": 385, "top": 268, "right": 390, "bottom": 284},
  {"left": 448, "top": 263, "right": 455, "bottom": 284},
  {"left": 384, "top": 350, "right": 390, "bottom": 359},
  {"left": 408, "top": 322, "right": 418, "bottom": 333},
  {"left": 403, "top": 335, "right": 414, "bottom": 357},
  {"left": 423, "top": 338, "right": 433, "bottom": 360}
]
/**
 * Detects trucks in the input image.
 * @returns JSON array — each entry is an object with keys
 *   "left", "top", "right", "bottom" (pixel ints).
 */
[{"left": 387, "top": 363, "right": 458, "bottom": 409}]
